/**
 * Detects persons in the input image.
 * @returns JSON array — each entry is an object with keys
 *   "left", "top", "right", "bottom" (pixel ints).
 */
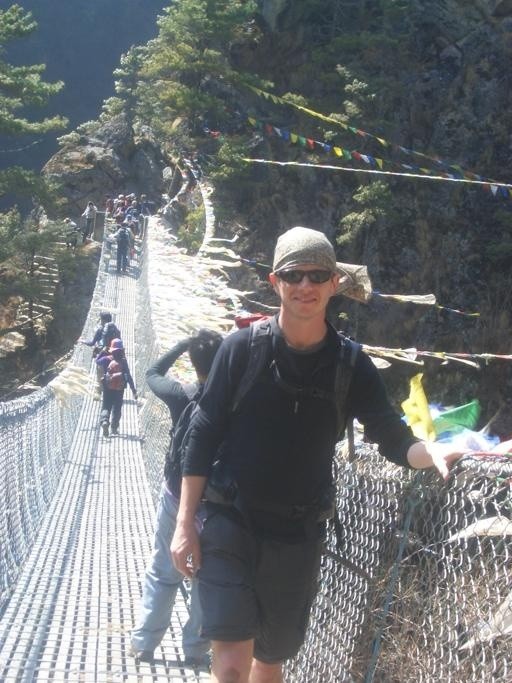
[
  {"left": 168, "top": 225, "right": 475, "bottom": 683},
  {"left": 92, "top": 338, "right": 140, "bottom": 439},
  {"left": 62, "top": 189, "right": 160, "bottom": 277},
  {"left": 127, "top": 329, "right": 226, "bottom": 668},
  {"left": 77, "top": 309, "right": 121, "bottom": 401}
]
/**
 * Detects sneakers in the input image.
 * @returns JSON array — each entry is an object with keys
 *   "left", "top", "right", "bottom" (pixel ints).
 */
[{"left": 102, "top": 422, "right": 109, "bottom": 437}]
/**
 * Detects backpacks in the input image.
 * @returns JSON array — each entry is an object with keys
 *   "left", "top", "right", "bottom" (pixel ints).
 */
[
  {"left": 106, "top": 354, "right": 125, "bottom": 390},
  {"left": 164, "top": 382, "right": 199, "bottom": 500}
]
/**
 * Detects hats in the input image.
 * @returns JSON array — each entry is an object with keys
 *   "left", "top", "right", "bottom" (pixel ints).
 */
[{"left": 108, "top": 338, "right": 124, "bottom": 352}]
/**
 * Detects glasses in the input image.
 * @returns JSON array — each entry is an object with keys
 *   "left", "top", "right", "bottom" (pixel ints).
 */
[{"left": 279, "top": 270, "right": 331, "bottom": 284}]
